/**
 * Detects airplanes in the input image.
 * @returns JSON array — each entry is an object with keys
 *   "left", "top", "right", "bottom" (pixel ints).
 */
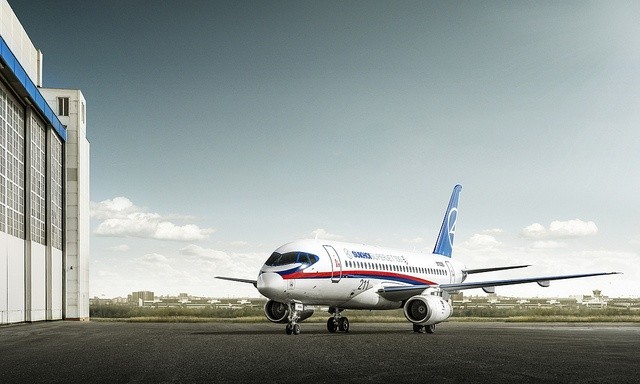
[{"left": 214, "top": 183, "right": 625, "bottom": 335}]
